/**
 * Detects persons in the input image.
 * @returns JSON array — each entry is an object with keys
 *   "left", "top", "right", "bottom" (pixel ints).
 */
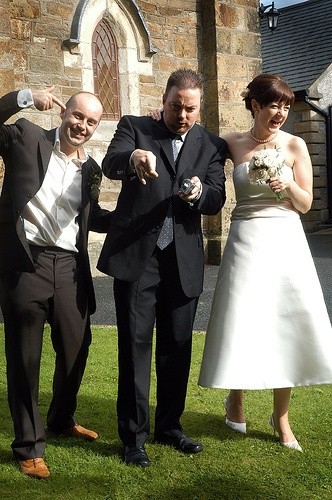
[
  {"left": 197, "top": 73, "right": 332, "bottom": 453},
  {"left": 95, "top": 69, "right": 226, "bottom": 466},
  {"left": 0, "top": 86, "right": 111, "bottom": 479}
]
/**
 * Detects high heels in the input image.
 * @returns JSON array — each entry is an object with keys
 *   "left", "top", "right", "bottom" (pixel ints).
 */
[
  {"left": 224, "top": 396, "right": 247, "bottom": 433},
  {"left": 270, "top": 414, "right": 303, "bottom": 453}
]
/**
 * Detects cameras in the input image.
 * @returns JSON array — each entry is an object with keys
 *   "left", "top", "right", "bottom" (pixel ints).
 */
[{"left": 179, "top": 178, "right": 196, "bottom": 195}]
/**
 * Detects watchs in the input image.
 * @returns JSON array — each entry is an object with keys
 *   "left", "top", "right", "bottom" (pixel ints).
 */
[{"left": 191, "top": 183, "right": 203, "bottom": 203}]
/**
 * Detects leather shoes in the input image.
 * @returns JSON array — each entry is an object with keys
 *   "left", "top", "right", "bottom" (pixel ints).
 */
[
  {"left": 124, "top": 445, "right": 150, "bottom": 468},
  {"left": 152, "top": 433, "right": 203, "bottom": 455}
]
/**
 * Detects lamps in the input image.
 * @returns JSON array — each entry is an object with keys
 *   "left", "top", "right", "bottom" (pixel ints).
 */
[{"left": 258, "top": 2, "right": 281, "bottom": 35}]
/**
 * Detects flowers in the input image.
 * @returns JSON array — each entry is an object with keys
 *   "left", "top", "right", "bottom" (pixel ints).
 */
[
  {"left": 78, "top": 164, "right": 101, "bottom": 199},
  {"left": 248, "top": 145, "right": 284, "bottom": 201},
  {"left": 240, "top": 88, "right": 250, "bottom": 101}
]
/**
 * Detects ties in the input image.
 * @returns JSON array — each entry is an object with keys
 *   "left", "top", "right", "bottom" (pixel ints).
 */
[{"left": 156, "top": 134, "right": 180, "bottom": 250}]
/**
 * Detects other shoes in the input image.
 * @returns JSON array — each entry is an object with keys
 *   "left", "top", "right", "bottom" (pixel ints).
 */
[
  {"left": 18, "top": 458, "right": 51, "bottom": 479},
  {"left": 63, "top": 423, "right": 99, "bottom": 440}
]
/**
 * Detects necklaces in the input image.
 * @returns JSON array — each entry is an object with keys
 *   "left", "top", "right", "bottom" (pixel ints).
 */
[{"left": 250, "top": 126, "right": 278, "bottom": 143}]
